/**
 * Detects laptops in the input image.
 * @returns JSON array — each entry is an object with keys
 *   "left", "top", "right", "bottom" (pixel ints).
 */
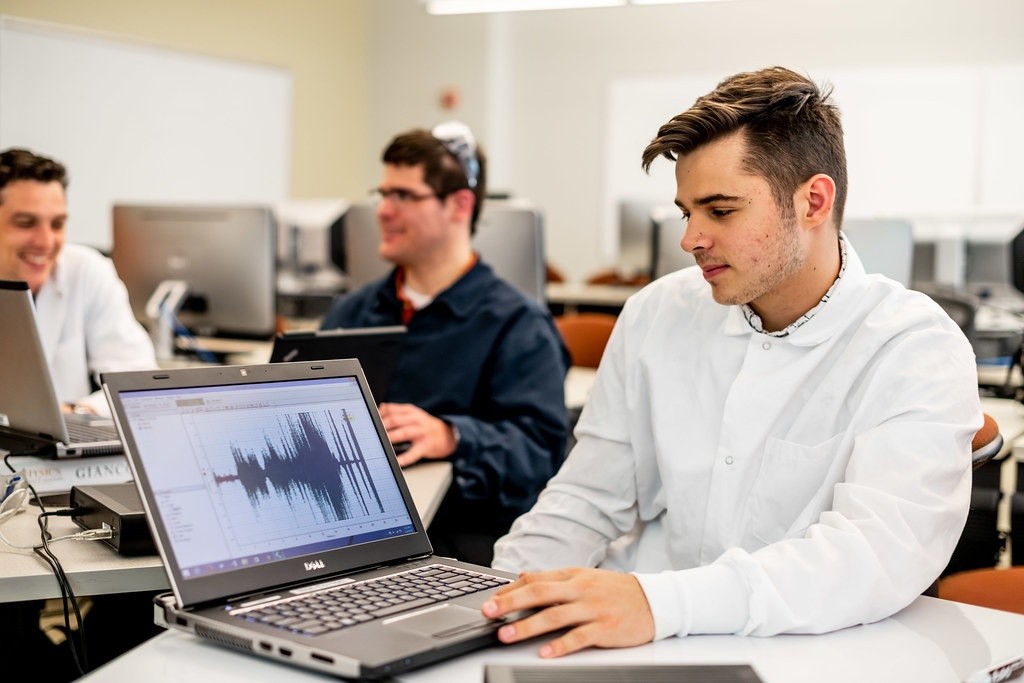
[
  {"left": 0, "top": 279, "right": 123, "bottom": 459},
  {"left": 100, "top": 357, "right": 554, "bottom": 682}
]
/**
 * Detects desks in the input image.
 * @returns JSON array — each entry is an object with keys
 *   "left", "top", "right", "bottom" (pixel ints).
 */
[
  {"left": 547, "top": 280, "right": 634, "bottom": 306},
  {"left": 69, "top": 594, "right": 1024, "bottom": 683},
  {"left": 0, "top": 450, "right": 174, "bottom": 603}
]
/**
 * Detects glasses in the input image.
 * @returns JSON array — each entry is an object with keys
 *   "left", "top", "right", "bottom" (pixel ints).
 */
[{"left": 369, "top": 188, "right": 440, "bottom": 206}]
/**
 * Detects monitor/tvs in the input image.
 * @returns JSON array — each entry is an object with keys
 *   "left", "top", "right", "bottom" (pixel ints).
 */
[
  {"left": 343, "top": 205, "right": 547, "bottom": 306},
  {"left": 113, "top": 204, "right": 278, "bottom": 341},
  {"left": 611, "top": 203, "right": 1024, "bottom": 394}
]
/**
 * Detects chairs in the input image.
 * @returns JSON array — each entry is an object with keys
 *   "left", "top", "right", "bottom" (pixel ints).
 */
[
  {"left": 938, "top": 566, "right": 1024, "bottom": 614},
  {"left": 556, "top": 314, "right": 616, "bottom": 368}
]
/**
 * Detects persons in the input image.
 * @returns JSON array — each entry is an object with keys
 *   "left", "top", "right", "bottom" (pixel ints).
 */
[
  {"left": 482, "top": 64, "right": 986, "bottom": 659},
  {"left": 314, "top": 123, "right": 573, "bottom": 564},
  {"left": 1, "top": 144, "right": 165, "bottom": 683}
]
